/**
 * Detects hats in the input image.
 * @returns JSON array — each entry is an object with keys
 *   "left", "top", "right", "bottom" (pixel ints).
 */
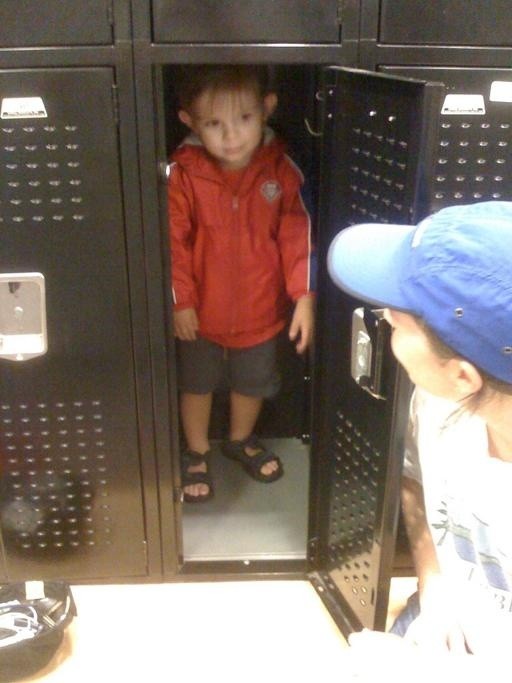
[{"left": 325, "top": 198, "right": 512, "bottom": 385}]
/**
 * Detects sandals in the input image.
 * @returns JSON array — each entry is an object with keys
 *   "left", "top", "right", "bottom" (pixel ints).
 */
[
  {"left": 181, "top": 437, "right": 215, "bottom": 503},
  {"left": 219, "top": 434, "right": 285, "bottom": 482}
]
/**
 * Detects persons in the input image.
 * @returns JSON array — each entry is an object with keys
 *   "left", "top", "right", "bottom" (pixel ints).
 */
[
  {"left": 168, "top": 65, "right": 316, "bottom": 504},
  {"left": 327, "top": 198, "right": 512, "bottom": 662}
]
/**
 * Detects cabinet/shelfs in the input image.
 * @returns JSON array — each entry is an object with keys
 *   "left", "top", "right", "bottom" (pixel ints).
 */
[{"left": 0, "top": 0, "right": 512, "bottom": 647}]
